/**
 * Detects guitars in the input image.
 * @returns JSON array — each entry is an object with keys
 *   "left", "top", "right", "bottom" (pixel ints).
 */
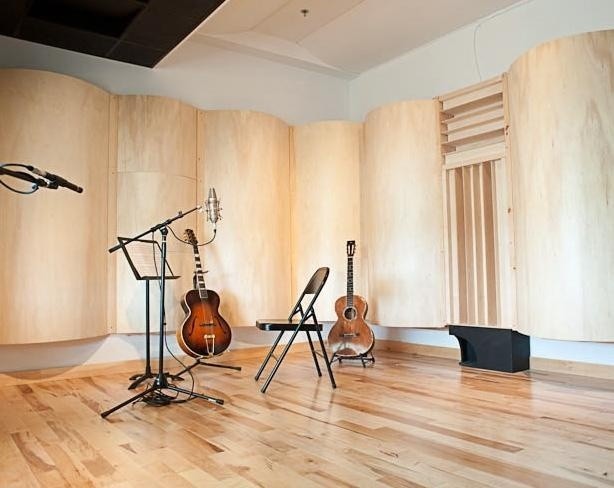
[
  {"left": 327, "top": 240, "right": 375, "bottom": 358},
  {"left": 176, "top": 229, "right": 232, "bottom": 359}
]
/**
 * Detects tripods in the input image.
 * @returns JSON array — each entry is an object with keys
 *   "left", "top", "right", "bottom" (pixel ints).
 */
[
  {"left": 99, "top": 230, "right": 224, "bottom": 421},
  {"left": 128, "top": 281, "right": 184, "bottom": 389}
]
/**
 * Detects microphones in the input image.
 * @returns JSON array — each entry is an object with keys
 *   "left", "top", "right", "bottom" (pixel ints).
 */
[
  {"left": 27, "top": 162, "right": 85, "bottom": 196},
  {"left": 201, "top": 185, "right": 224, "bottom": 224}
]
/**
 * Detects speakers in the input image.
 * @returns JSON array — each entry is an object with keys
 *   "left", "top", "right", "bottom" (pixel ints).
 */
[{"left": 446, "top": 324, "right": 531, "bottom": 373}]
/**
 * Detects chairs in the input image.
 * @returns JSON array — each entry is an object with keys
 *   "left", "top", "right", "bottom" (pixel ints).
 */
[{"left": 254, "top": 266, "right": 336, "bottom": 393}]
